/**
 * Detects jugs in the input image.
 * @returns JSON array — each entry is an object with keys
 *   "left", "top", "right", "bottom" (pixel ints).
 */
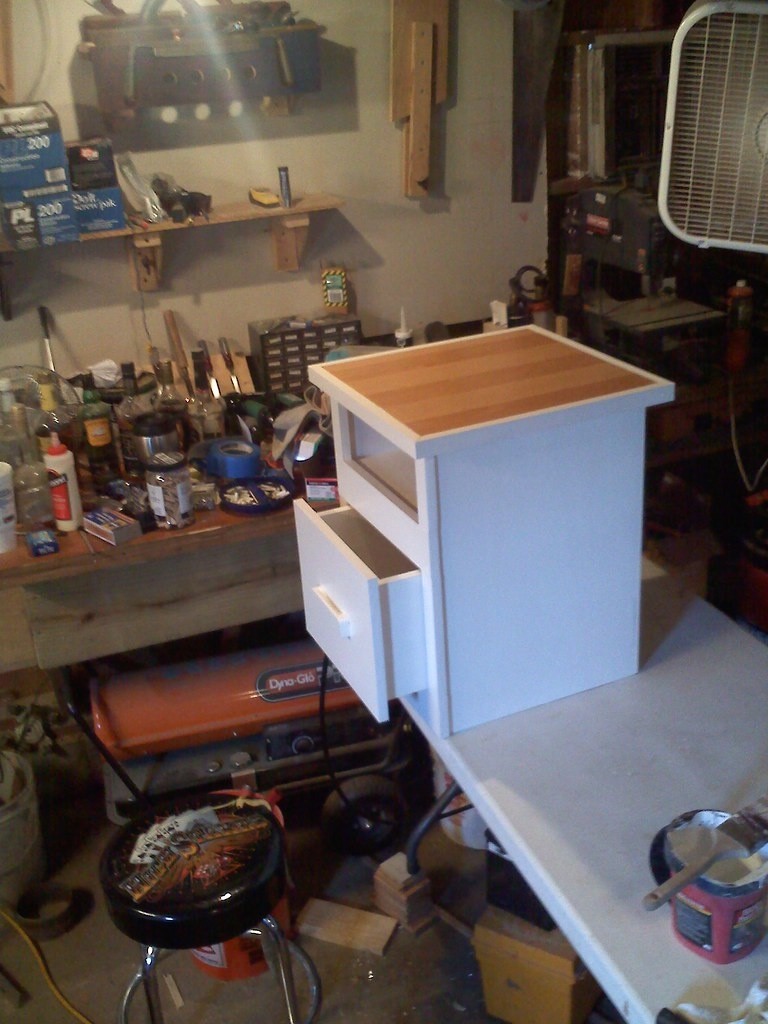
[{"left": 130, "top": 409, "right": 196, "bottom": 466}]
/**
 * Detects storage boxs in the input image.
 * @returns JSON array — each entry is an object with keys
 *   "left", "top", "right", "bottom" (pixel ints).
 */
[
  {"left": 0, "top": 100, "right": 125, "bottom": 251},
  {"left": 25, "top": 529, "right": 59, "bottom": 557},
  {"left": 248, "top": 314, "right": 363, "bottom": 395},
  {"left": 82, "top": 507, "right": 143, "bottom": 547},
  {"left": 470, "top": 828, "right": 605, "bottom": 1024},
  {"left": 304, "top": 478, "right": 338, "bottom": 501}
]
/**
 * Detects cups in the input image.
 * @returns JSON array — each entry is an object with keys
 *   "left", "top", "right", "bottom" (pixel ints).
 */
[
  {"left": 0, "top": 462, "right": 20, "bottom": 554},
  {"left": 665, "top": 810, "right": 768, "bottom": 963}
]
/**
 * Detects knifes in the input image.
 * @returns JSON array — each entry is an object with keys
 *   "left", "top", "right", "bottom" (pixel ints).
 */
[
  {"left": 218, "top": 337, "right": 242, "bottom": 395},
  {"left": 199, "top": 340, "right": 223, "bottom": 401}
]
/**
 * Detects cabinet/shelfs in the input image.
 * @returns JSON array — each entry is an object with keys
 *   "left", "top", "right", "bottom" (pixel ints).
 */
[{"left": 293, "top": 323, "right": 676, "bottom": 739}]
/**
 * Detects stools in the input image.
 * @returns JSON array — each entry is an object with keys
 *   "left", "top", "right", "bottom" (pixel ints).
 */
[{"left": 99, "top": 794, "right": 323, "bottom": 1024}]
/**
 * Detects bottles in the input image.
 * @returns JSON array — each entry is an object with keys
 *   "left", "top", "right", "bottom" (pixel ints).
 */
[
  {"left": 0, "top": 377, "right": 40, "bottom": 463},
  {"left": 152, "top": 359, "right": 193, "bottom": 456},
  {"left": 187, "top": 352, "right": 227, "bottom": 446},
  {"left": 395, "top": 306, "right": 413, "bottom": 350},
  {"left": 42, "top": 431, "right": 84, "bottom": 531},
  {"left": 115, "top": 360, "right": 143, "bottom": 487},
  {"left": 506, "top": 277, "right": 530, "bottom": 328},
  {"left": 77, "top": 370, "right": 127, "bottom": 499},
  {"left": 12, "top": 403, "right": 54, "bottom": 535},
  {"left": 726, "top": 279, "right": 754, "bottom": 374},
  {"left": 36, "top": 371, "right": 82, "bottom": 485},
  {"left": 532, "top": 275, "right": 556, "bottom": 331}
]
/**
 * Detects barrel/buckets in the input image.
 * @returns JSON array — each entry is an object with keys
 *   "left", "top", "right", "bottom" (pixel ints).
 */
[{"left": 0, "top": 752, "right": 47, "bottom": 911}]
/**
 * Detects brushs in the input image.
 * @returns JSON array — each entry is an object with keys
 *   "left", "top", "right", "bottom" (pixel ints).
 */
[{"left": 643, "top": 797, "right": 768, "bottom": 912}]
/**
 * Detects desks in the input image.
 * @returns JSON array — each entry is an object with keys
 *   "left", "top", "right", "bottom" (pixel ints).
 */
[
  {"left": 395, "top": 554, "right": 767, "bottom": 1024},
  {"left": 0, "top": 461, "right": 338, "bottom": 672}
]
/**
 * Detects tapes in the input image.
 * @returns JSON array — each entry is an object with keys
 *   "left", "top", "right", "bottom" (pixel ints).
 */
[{"left": 208, "top": 438, "right": 262, "bottom": 479}]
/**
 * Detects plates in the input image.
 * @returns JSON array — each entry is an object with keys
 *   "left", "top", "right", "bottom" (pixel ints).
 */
[{"left": 220, "top": 475, "right": 296, "bottom": 513}]
